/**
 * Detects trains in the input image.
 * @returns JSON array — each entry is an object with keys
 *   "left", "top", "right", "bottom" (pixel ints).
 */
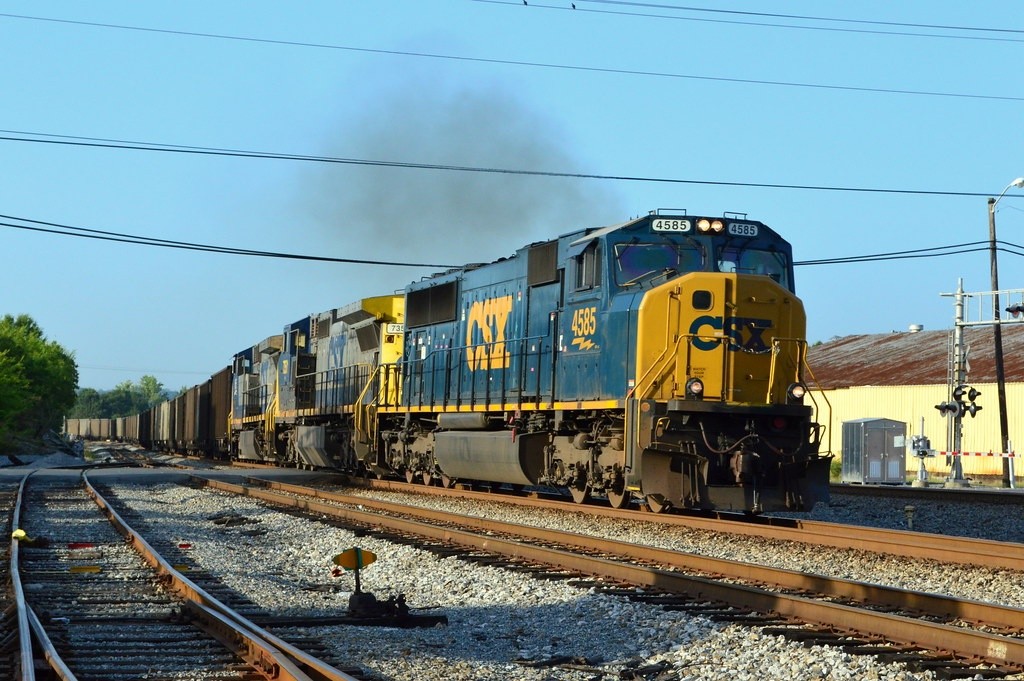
[{"left": 61, "top": 208, "right": 836, "bottom": 523}]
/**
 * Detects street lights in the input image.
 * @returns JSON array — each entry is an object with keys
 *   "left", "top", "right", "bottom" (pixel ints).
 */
[{"left": 987, "top": 177, "right": 1024, "bottom": 488}]
